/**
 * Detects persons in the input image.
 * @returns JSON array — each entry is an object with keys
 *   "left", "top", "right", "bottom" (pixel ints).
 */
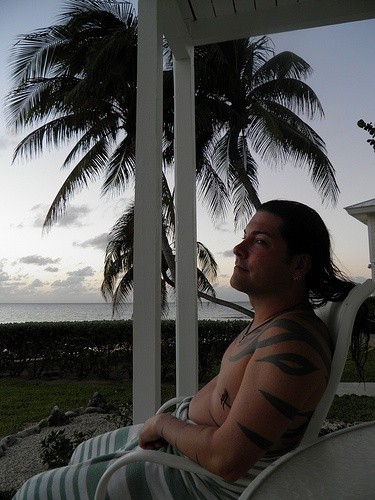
[{"left": 12, "top": 199, "right": 374, "bottom": 499}]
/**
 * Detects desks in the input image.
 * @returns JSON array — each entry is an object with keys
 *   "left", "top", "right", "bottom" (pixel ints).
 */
[{"left": 237, "top": 419, "right": 375, "bottom": 500}]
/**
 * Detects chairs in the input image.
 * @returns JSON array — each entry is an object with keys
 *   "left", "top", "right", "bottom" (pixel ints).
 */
[{"left": 93, "top": 276, "right": 375, "bottom": 499}]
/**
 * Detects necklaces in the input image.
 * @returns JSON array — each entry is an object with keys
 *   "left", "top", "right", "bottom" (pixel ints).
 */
[{"left": 238, "top": 302, "right": 305, "bottom": 344}]
